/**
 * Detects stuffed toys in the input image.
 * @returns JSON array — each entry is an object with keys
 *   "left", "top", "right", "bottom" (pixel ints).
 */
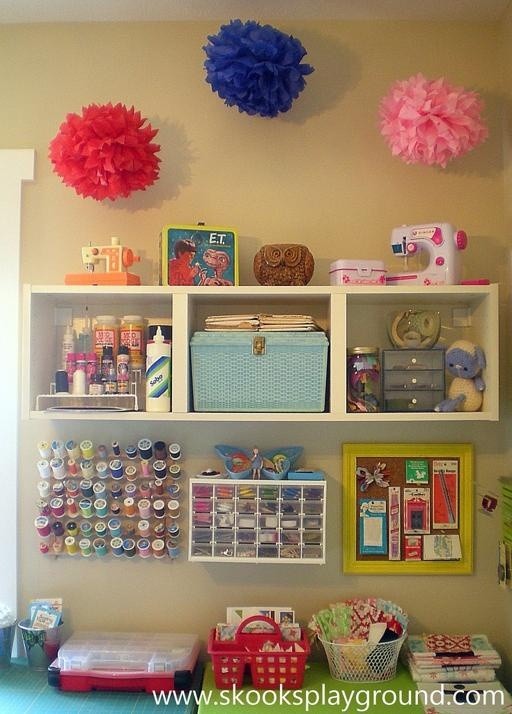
[
  {"left": 434, "top": 341, "right": 485, "bottom": 412},
  {"left": 255, "top": 244, "right": 312, "bottom": 285}
]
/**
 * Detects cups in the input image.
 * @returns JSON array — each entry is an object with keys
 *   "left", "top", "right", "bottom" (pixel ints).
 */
[
  {"left": 18, "top": 617, "right": 65, "bottom": 671},
  {"left": 0, "top": 622, "right": 17, "bottom": 667}
]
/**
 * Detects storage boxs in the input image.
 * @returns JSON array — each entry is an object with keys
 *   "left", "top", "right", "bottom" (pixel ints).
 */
[
  {"left": 47, "top": 630, "right": 204, "bottom": 698},
  {"left": 330, "top": 259, "right": 388, "bottom": 286},
  {"left": 380, "top": 345, "right": 447, "bottom": 411}
]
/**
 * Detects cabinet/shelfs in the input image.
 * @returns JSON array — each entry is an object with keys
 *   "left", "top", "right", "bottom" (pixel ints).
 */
[
  {"left": 35, "top": 368, "right": 143, "bottom": 411},
  {"left": 21, "top": 285, "right": 501, "bottom": 421}
]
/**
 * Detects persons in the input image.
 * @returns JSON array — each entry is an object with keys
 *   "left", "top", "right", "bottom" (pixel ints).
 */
[
  {"left": 249, "top": 446, "right": 265, "bottom": 480},
  {"left": 169, "top": 239, "right": 199, "bottom": 285}
]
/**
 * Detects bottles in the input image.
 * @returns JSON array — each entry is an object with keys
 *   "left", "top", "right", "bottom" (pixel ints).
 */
[
  {"left": 49, "top": 312, "right": 171, "bottom": 412},
  {"left": 346, "top": 346, "right": 382, "bottom": 412}
]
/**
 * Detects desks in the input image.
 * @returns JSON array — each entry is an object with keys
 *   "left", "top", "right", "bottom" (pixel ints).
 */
[
  {"left": 197, "top": 660, "right": 512, "bottom": 714},
  {"left": 2, "top": 657, "right": 204, "bottom": 712}
]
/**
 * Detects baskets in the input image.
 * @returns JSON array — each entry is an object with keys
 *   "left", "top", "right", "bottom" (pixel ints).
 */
[{"left": 207, "top": 614, "right": 311, "bottom": 690}]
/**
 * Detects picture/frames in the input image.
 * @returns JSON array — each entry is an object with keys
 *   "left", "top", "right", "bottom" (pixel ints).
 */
[
  {"left": 340, "top": 443, "right": 475, "bottom": 577},
  {"left": 162, "top": 223, "right": 240, "bottom": 287}
]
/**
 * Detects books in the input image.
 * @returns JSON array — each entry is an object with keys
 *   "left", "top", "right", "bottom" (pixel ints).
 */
[
  {"left": 413, "top": 683, "right": 512, "bottom": 714},
  {"left": 401, "top": 632, "right": 502, "bottom": 682}
]
